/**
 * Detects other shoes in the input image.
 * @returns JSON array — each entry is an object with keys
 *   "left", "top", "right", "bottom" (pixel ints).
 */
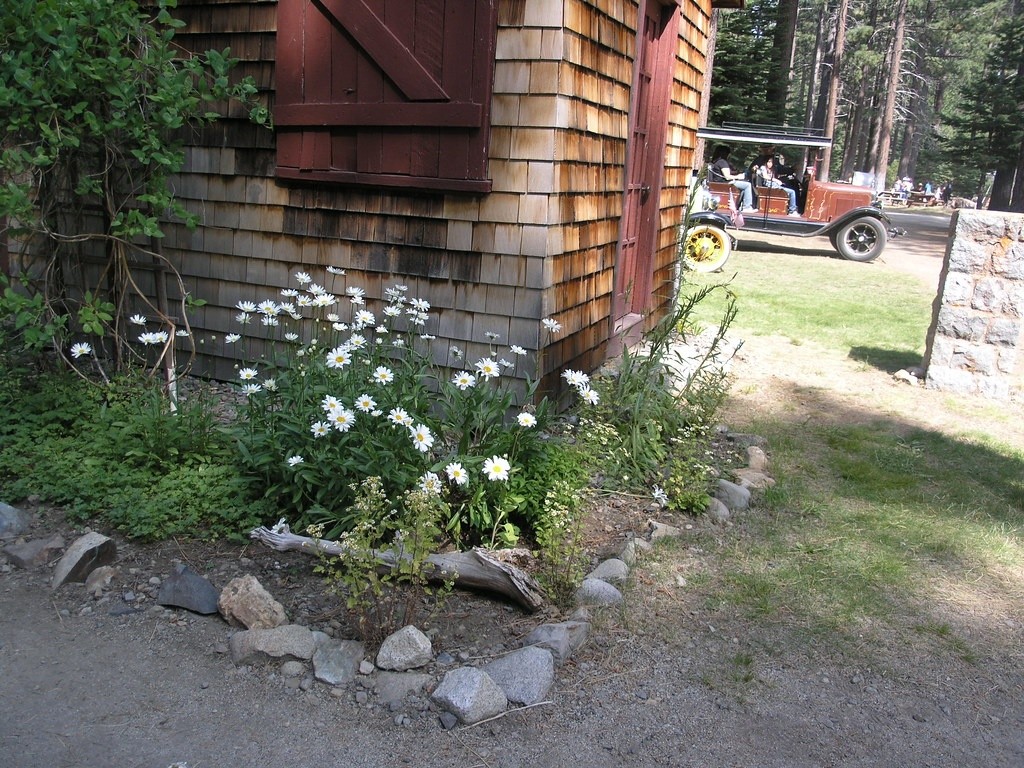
[
  {"left": 788, "top": 211, "right": 801, "bottom": 219},
  {"left": 740, "top": 206, "right": 758, "bottom": 213}
]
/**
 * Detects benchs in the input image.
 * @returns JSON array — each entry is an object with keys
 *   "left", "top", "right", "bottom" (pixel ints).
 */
[
  {"left": 751, "top": 169, "right": 791, "bottom": 215},
  {"left": 707, "top": 162, "right": 741, "bottom": 211},
  {"left": 875, "top": 196, "right": 907, "bottom": 200},
  {"left": 906, "top": 200, "right": 931, "bottom": 208}
]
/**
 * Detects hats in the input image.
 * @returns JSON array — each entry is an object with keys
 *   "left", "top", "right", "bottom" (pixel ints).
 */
[{"left": 758, "top": 144, "right": 775, "bottom": 150}]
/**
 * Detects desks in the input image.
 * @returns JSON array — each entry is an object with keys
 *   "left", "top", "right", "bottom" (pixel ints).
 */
[
  {"left": 912, "top": 195, "right": 933, "bottom": 207},
  {"left": 882, "top": 191, "right": 906, "bottom": 206}
]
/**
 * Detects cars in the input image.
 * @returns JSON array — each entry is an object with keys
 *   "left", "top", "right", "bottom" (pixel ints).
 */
[{"left": 679, "top": 120, "right": 894, "bottom": 274}]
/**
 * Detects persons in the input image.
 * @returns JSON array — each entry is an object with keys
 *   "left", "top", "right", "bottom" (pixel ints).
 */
[
  {"left": 712, "top": 144, "right": 758, "bottom": 213},
  {"left": 750, "top": 145, "right": 800, "bottom": 215},
  {"left": 892, "top": 176, "right": 952, "bottom": 205}
]
